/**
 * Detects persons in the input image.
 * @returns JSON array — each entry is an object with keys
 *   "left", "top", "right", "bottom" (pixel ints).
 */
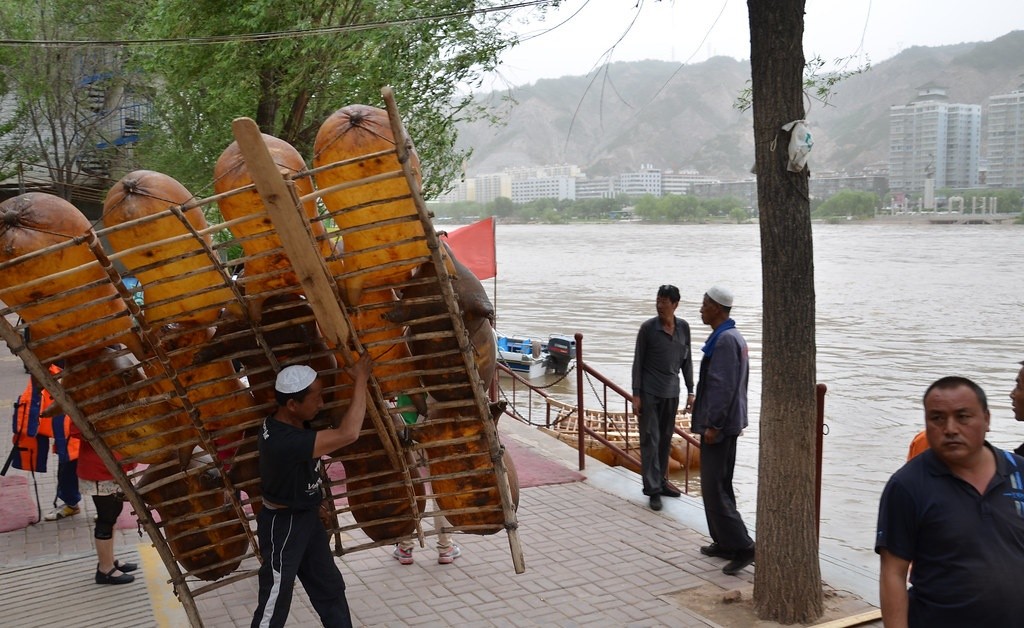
[
  {"left": 44, "top": 415, "right": 82, "bottom": 520},
  {"left": 630, "top": 284, "right": 695, "bottom": 510},
  {"left": 78, "top": 433, "right": 138, "bottom": 583},
  {"left": 391, "top": 384, "right": 461, "bottom": 564},
  {"left": 1008, "top": 361, "right": 1024, "bottom": 457},
  {"left": 689, "top": 287, "right": 757, "bottom": 576},
  {"left": 874, "top": 376, "right": 1024, "bottom": 628},
  {"left": 251, "top": 351, "right": 373, "bottom": 628}
]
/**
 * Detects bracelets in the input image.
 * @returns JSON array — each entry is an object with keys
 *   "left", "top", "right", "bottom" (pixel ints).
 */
[{"left": 688, "top": 393, "right": 695, "bottom": 397}]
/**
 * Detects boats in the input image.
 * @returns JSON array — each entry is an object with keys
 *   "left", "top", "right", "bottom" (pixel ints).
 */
[
  {"left": 495, "top": 329, "right": 577, "bottom": 380},
  {"left": 538, "top": 397, "right": 701, "bottom": 472}
]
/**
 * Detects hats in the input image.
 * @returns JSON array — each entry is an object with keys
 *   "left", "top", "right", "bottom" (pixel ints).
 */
[
  {"left": 276, "top": 366, "right": 317, "bottom": 394},
  {"left": 707, "top": 285, "right": 735, "bottom": 307}
]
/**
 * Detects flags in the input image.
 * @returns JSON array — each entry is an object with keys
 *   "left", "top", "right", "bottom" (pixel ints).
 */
[{"left": 438, "top": 217, "right": 497, "bottom": 280}]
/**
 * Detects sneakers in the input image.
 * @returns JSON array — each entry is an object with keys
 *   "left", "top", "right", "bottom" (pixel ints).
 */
[
  {"left": 700, "top": 542, "right": 731, "bottom": 560},
  {"left": 722, "top": 542, "right": 756, "bottom": 574},
  {"left": 44, "top": 504, "right": 81, "bottom": 521},
  {"left": 393, "top": 546, "right": 414, "bottom": 565},
  {"left": 438, "top": 543, "right": 461, "bottom": 564}
]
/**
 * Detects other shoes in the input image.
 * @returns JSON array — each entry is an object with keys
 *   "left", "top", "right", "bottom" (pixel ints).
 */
[
  {"left": 95, "top": 559, "right": 137, "bottom": 585},
  {"left": 650, "top": 487, "right": 681, "bottom": 511}
]
